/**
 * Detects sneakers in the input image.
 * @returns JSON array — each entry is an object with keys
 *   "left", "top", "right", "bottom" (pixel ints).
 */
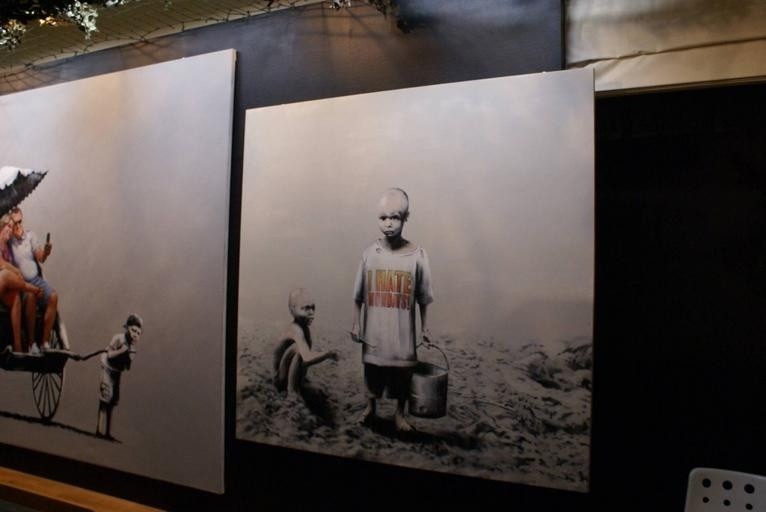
[
  {"left": 95, "top": 432, "right": 114, "bottom": 440},
  {"left": 29, "top": 341, "right": 50, "bottom": 354}
]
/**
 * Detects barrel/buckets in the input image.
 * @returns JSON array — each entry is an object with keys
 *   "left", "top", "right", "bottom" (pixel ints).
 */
[{"left": 408, "top": 342, "right": 450, "bottom": 420}]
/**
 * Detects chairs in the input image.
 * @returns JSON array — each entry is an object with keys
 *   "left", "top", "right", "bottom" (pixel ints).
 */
[{"left": 683, "top": 466, "right": 766, "bottom": 512}]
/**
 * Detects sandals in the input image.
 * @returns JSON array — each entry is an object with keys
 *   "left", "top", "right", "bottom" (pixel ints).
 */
[{"left": 32, "top": 285, "right": 45, "bottom": 299}]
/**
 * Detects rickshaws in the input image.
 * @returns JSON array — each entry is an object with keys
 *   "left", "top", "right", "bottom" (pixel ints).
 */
[{"left": 0, "top": 166, "right": 136, "bottom": 420}]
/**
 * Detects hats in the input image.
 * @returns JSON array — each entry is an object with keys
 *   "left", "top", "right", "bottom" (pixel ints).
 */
[{"left": 123, "top": 314, "right": 143, "bottom": 329}]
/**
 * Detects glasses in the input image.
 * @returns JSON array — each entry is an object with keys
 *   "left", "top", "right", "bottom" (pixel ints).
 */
[{"left": 15, "top": 219, "right": 22, "bottom": 225}]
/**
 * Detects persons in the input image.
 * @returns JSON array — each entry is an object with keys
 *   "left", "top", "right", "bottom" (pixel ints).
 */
[
  {"left": 6, "top": 206, "right": 58, "bottom": 356},
  {"left": 266, "top": 285, "right": 340, "bottom": 406},
  {"left": 347, "top": 188, "right": 434, "bottom": 439},
  {"left": 90, "top": 312, "right": 145, "bottom": 445},
  {"left": 0, "top": 213, "right": 42, "bottom": 359}
]
